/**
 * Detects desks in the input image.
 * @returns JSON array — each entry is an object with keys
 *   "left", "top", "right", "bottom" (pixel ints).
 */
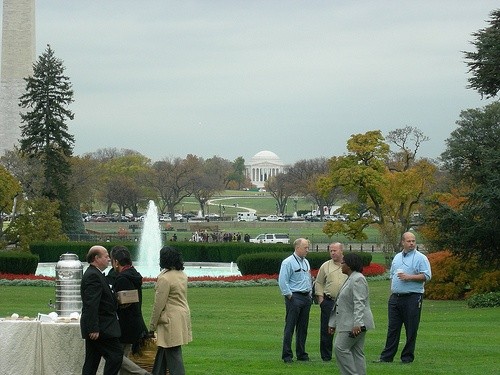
[{"left": 0, "top": 314, "right": 108, "bottom": 375}]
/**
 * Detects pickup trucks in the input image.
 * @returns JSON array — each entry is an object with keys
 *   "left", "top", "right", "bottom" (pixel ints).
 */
[
  {"left": 249, "top": 233, "right": 290, "bottom": 245},
  {"left": 259, "top": 215, "right": 284, "bottom": 222}
]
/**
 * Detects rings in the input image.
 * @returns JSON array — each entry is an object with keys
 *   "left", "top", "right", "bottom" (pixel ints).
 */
[{"left": 358, "top": 331, "right": 359, "bottom": 333}]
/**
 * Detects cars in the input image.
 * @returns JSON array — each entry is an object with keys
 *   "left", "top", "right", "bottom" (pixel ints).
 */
[
  {"left": 82, "top": 211, "right": 181, "bottom": 222},
  {"left": 306, "top": 214, "right": 380, "bottom": 224}
]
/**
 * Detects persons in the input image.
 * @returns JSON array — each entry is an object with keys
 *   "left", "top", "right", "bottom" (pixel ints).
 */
[
  {"left": 371, "top": 232, "right": 432, "bottom": 363},
  {"left": 80, "top": 245, "right": 124, "bottom": 375},
  {"left": 109, "top": 244, "right": 148, "bottom": 375},
  {"left": 148, "top": 245, "right": 192, "bottom": 375},
  {"left": 314, "top": 242, "right": 349, "bottom": 361},
  {"left": 327, "top": 253, "right": 375, "bottom": 375},
  {"left": 104, "top": 245, "right": 128, "bottom": 293},
  {"left": 97, "top": 229, "right": 251, "bottom": 243},
  {"left": 278, "top": 238, "right": 313, "bottom": 363}
]
